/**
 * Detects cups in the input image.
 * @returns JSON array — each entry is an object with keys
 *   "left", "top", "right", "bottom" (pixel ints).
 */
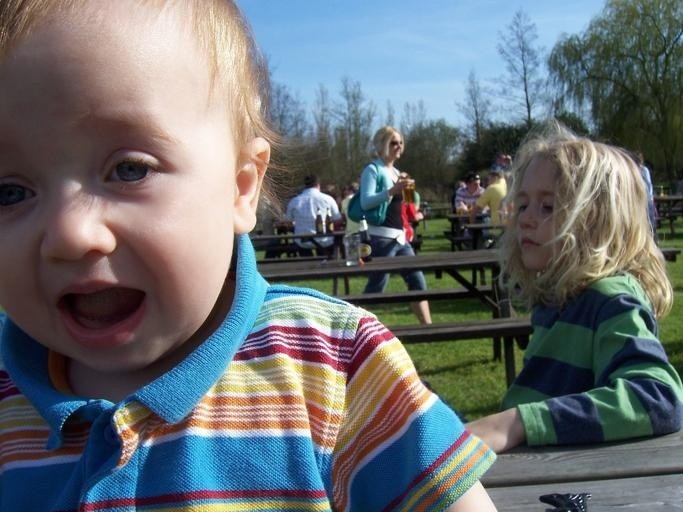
[
  {"left": 342, "top": 235, "right": 360, "bottom": 267},
  {"left": 401, "top": 179, "right": 415, "bottom": 204},
  {"left": 456, "top": 205, "right": 464, "bottom": 216}
]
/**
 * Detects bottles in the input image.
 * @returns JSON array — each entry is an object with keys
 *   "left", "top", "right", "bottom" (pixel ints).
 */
[
  {"left": 315, "top": 207, "right": 335, "bottom": 234},
  {"left": 358, "top": 216, "right": 372, "bottom": 262}
]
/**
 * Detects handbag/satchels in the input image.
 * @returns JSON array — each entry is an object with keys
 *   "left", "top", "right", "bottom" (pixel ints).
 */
[{"left": 348, "top": 191, "right": 388, "bottom": 226}]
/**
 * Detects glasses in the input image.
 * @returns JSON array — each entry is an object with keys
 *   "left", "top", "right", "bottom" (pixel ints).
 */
[{"left": 391, "top": 141, "right": 402, "bottom": 145}]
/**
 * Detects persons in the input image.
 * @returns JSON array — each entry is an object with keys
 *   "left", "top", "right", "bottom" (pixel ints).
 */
[
  {"left": 357, "top": 124, "right": 434, "bottom": 329},
  {"left": 250, "top": 172, "right": 433, "bottom": 261},
  {"left": 0, "top": 1, "right": 499, "bottom": 511},
  {"left": 445, "top": 150, "right": 683, "bottom": 249},
  {"left": 464, "top": 119, "right": 683, "bottom": 457}
]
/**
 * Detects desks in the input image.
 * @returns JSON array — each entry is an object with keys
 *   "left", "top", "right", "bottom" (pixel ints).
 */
[{"left": 251, "top": 185, "right": 682, "bottom": 511}]
